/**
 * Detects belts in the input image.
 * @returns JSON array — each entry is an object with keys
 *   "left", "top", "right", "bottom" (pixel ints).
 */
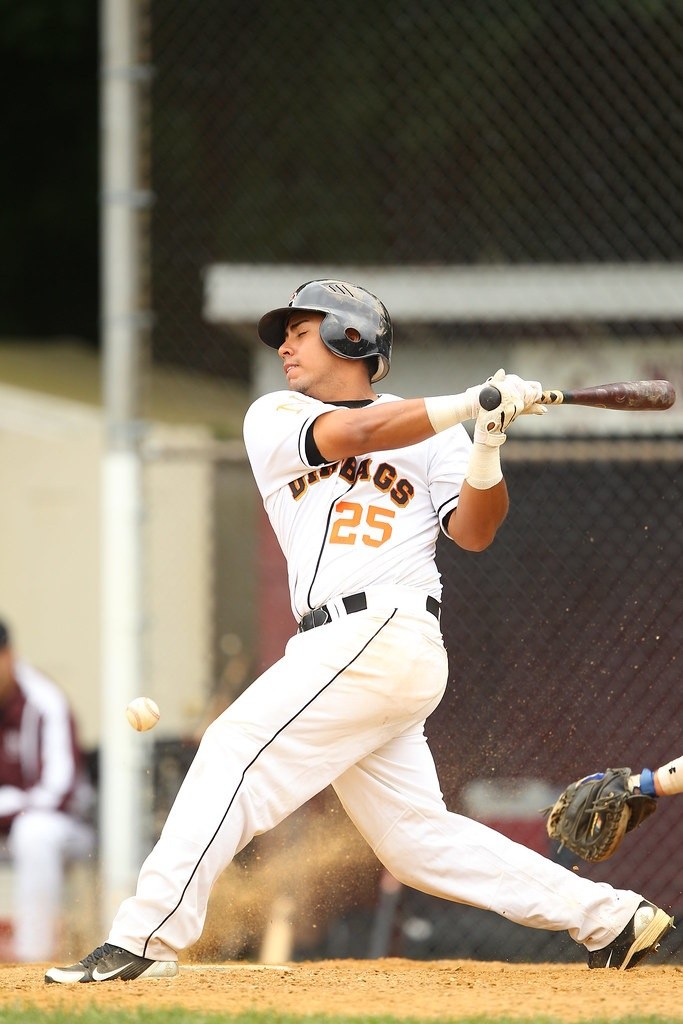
[{"left": 298, "top": 592, "right": 440, "bottom": 634}]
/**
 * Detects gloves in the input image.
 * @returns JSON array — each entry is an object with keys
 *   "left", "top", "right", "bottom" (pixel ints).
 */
[
  {"left": 464, "top": 368, "right": 526, "bottom": 490},
  {"left": 422, "top": 372, "right": 549, "bottom": 435}
]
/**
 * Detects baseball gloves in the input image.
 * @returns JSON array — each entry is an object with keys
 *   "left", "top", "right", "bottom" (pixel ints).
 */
[{"left": 539, "top": 768, "right": 659, "bottom": 863}]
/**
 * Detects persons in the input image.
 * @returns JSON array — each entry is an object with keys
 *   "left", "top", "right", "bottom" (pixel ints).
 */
[
  {"left": 45, "top": 277, "right": 678, "bottom": 983},
  {"left": 547, "top": 755, "right": 683, "bottom": 863},
  {"left": 0, "top": 618, "right": 100, "bottom": 963}
]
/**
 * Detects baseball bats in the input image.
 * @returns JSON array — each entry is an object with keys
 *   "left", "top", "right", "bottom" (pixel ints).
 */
[{"left": 479, "top": 376, "right": 677, "bottom": 413}]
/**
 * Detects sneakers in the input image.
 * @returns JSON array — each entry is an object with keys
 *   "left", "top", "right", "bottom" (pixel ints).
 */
[
  {"left": 42, "top": 941, "right": 181, "bottom": 985},
  {"left": 586, "top": 901, "right": 675, "bottom": 970}
]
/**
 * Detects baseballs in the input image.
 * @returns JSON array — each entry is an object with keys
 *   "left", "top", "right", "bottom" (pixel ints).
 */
[{"left": 126, "top": 697, "right": 160, "bottom": 732}]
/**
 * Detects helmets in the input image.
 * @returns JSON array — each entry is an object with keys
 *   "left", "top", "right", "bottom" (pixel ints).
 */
[{"left": 259, "top": 281, "right": 395, "bottom": 384}]
